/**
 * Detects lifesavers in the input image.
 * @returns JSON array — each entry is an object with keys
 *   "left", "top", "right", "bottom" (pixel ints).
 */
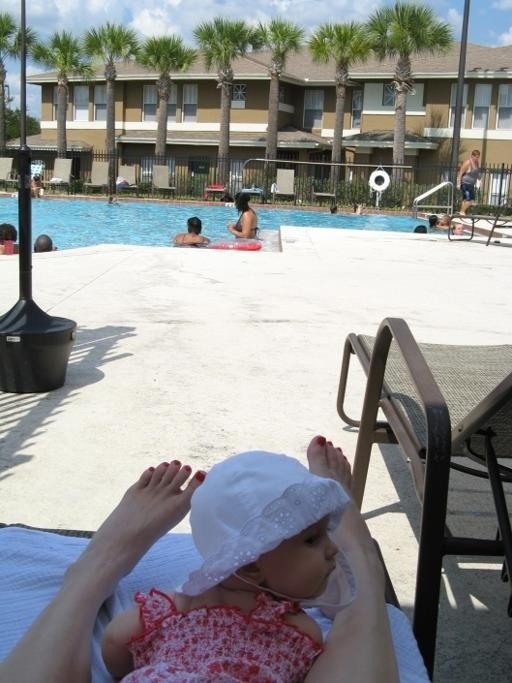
[
  {"left": 369, "top": 170, "right": 390, "bottom": 191},
  {"left": 207, "top": 238, "right": 262, "bottom": 251}
]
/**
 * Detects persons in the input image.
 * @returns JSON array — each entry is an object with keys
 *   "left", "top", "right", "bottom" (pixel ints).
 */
[
  {"left": 429, "top": 215, "right": 438, "bottom": 229},
  {"left": 330, "top": 205, "right": 338, "bottom": 214},
  {"left": 0, "top": 223, "right": 19, "bottom": 254},
  {"left": 456, "top": 149, "right": 481, "bottom": 215},
  {"left": 228, "top": 192, "right": 257, "bottom": 238},
  {"left": 34, "top": 234, "right": 57, "bottom": 252},
  {"left": 414, "top": 225, "right": 427, "bottom": 233},
  {"left": 112, "top": 196, "right": 120, "bottom": 206},
  {"left": 353, "top": 203, "right": 363, "bottom": 215},
  {"left": 436, "top": 215, "right": 453, "bottom": 230},
  {"left": 30, "top": 176, "right": 44, "bottom": 198},
  {"left": 451, "top": 223, "right": 464, "bottom": 235},
  {"left": 175, "top": 218, "right": 210, "bottom": 247},
  {"left": 0, "top": 435, "right": 400, "bottom": 682},
  {"left": 108, "top": 197, "right": 114, "bottom": 204},
  {"left": 100, "top": 450, "right": 350, "bottom": 682}
]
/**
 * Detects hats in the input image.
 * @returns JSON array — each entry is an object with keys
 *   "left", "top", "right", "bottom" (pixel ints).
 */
[{"left": 177, "top": 450, "right": 351, "bottom": 597}]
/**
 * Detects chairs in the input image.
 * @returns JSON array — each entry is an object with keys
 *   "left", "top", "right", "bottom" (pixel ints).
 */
[
  {"left": 205, "top": 168, "right": 336, "bottom": 204},
  {"left": 335, "top": 316, "right": 511, "bottom": 683},
  {"left": 0, "top": 157, "right": 176, "bottom": 199}
]
